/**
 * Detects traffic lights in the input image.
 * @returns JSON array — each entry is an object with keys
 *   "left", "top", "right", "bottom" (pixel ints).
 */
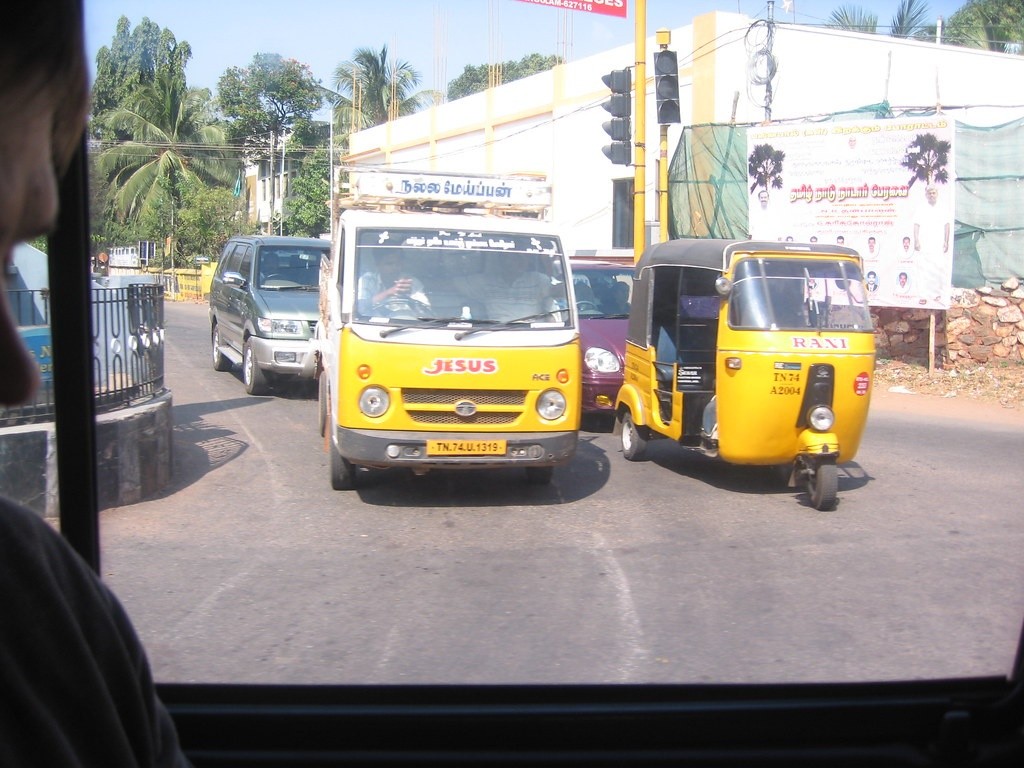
[
  {"left": 654, "top": 50, "right": 682, "bottom": 125},
  {"left": 601, "top": 68, "right": 632, "bottom": 166}
]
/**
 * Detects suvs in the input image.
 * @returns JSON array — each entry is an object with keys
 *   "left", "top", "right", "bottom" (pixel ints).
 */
[{"left": 208, "top": 234, "right": 333, "bottom": 395}]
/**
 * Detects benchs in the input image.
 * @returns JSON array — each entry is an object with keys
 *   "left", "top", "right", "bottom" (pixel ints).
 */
[{"left": 654, "top": 317, "right": 717, "bottom": 390}]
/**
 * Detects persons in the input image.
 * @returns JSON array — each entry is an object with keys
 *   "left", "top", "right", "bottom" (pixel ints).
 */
[
  {"left": 837, "top": 236, "right": 844, "bottom": 245},
  {"left": 358, "top": 238, "right": 431, "bottom": 320},
  {"left": 599, "top": 281, "right": 631, "bottom": 314},
  {"left": 759, "top": 190, "right": 769, "bottom": 209},
  {"left": 866, "top": 238, "right": 876, "bottom": 252},
  {"left": 896, "top": 272, "right": 909, "bottom": 293},
  {"left": 0, "top": 0, "right": 186, "bottom": 768},
  {"left": 914, "top": 185, "right": 950, "bottom": 304},
  {"left": 484, "top": 255, "right": 562, "bottom": 323},
  {"left": 903, "top": 237, "right": 910, "bottom": 252},
  {"left": 263, "top": 253, "right": 286, "bottom": 280},
  {"left": 866, "top": 271, "right": 878, "bottom": 291}
]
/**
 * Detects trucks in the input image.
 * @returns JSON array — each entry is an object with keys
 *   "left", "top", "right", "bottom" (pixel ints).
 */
[{"left": 317, "top": 166, "right": 582, "bottom": 489}]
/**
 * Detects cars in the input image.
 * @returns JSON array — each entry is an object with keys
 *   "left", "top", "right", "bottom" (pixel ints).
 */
[{"left": 562, "top": 260, "right": 637, "bottom": 420}]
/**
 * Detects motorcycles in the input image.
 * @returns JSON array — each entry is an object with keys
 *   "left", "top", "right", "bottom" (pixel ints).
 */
[{"left": 613, "top": 238, "right": 877, "bottom": 513}]
